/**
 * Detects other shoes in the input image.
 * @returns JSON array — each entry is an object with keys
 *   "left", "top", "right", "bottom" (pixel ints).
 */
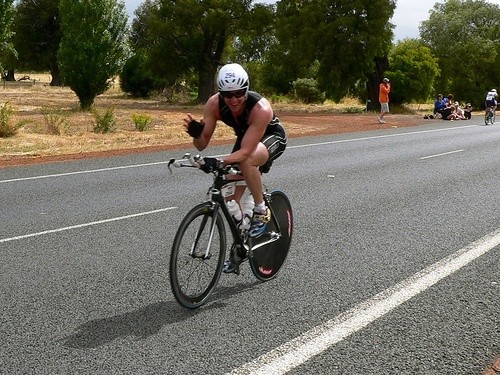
[
  {"left": 378, "top": 118, "right": 386, "bottom": 124},
  {"left": 249, "top": 206, "right": 271, "bottom": 239},
  {"left": 222, "top": 250, "right": 249, "bottom": 273}
]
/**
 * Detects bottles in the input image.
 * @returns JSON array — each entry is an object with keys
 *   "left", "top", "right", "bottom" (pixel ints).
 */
[
  {"left": 241, "top": 193, "right": 255, "bottom": 230},
  {"left": 227, "top": 198, "right": 246, "bottom": 230}
]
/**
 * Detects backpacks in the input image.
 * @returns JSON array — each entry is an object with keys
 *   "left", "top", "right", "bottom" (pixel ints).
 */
[{"left": 486, "top": 94, "right": 494, "bottom": 104}]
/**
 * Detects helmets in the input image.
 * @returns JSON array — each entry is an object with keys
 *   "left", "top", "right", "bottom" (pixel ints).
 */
[{"left": 216, "top": 63, "right": 249, "bottom": 97}]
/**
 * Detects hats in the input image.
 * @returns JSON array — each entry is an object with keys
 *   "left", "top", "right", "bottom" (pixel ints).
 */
[
  {"left": 467, "top": 103, "right": 471, "bottom": 106},
  {"left": 491, "top": 89, "right": 496, "bottom": 92},
  {"left": 383, "top": 78, "right": 389, "bottom": 82}
]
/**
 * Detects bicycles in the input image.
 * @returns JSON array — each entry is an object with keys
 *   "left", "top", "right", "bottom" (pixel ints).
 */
[
  {"left": 484, "top": 103, "right": 500, "bottom": 126},
  {"left": 167, "top": 152, "right": 294, "bottom": 311}
]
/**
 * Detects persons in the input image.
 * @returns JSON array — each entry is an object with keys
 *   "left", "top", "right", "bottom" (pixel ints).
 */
[
  {"left": 183, "top": 63, "right": 288, "bottom": 273},
  {"left": 378, "top": 78, "right": 390, "bottom": 123},
  {"left": 434, "top": 94, "right": 472, "bottom": 120},
  {"left": 486, "top": 89, "right": 498, "bottom": 117}
]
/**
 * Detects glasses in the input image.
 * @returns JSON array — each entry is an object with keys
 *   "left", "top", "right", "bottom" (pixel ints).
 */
[{"left": 220, "top": 88, "right": 247, "bottom": 98}]
